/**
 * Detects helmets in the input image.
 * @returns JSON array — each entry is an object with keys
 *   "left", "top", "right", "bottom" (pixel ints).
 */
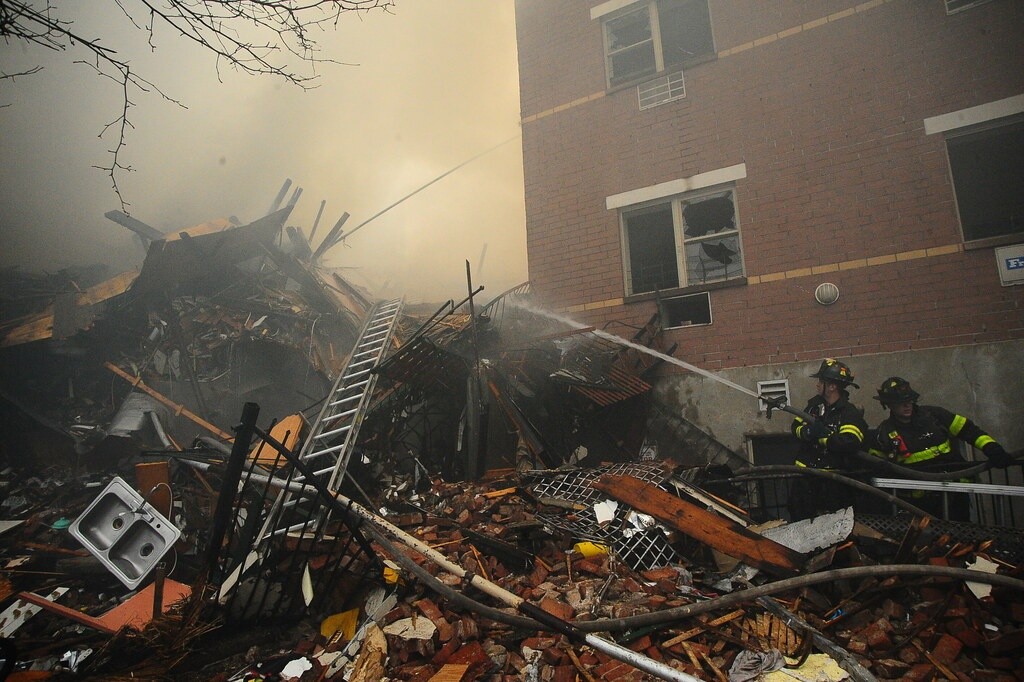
[
  {"left": 808, "top": 357, "right": 861, "bottom": 390},
  {"left": 872, "top": 377, "right": 921, "bottom": 400}
]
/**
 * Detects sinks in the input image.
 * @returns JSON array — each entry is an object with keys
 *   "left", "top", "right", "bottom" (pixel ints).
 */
[
  {"left": 67, "top": 477, "right": 145, "bottom": 554},
  {"left": 104, "top": 517, "right": 183, "bottom": 590}
]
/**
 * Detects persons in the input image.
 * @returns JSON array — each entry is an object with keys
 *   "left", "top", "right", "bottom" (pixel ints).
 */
[
  {"left": 870, "top": 377, "right": 1013, "bottom": 522},
  {"left": 792, "top": 356, "right": 868, "bottom": 471}
]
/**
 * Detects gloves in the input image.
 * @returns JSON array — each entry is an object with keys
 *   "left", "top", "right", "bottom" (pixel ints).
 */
[
  {"left": 983, "top": 441, "right": 1015, "bottom": 469},
  {"left": 806, "top": 421, "right": 827, "bottom": 438}
]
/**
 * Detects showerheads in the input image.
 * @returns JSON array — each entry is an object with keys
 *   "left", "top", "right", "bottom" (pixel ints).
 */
[{"left": 756, "top": 392, "right": 788, "bottom": 420}]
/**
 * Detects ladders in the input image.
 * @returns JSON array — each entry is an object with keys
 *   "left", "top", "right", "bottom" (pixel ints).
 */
[{"left": 249, "top": 295, "right": 406, "bottom": 537}]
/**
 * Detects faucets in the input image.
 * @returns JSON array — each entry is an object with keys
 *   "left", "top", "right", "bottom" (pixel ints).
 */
[{"left": 119, "top": 506, "right": 147, "bottom": 518}]
